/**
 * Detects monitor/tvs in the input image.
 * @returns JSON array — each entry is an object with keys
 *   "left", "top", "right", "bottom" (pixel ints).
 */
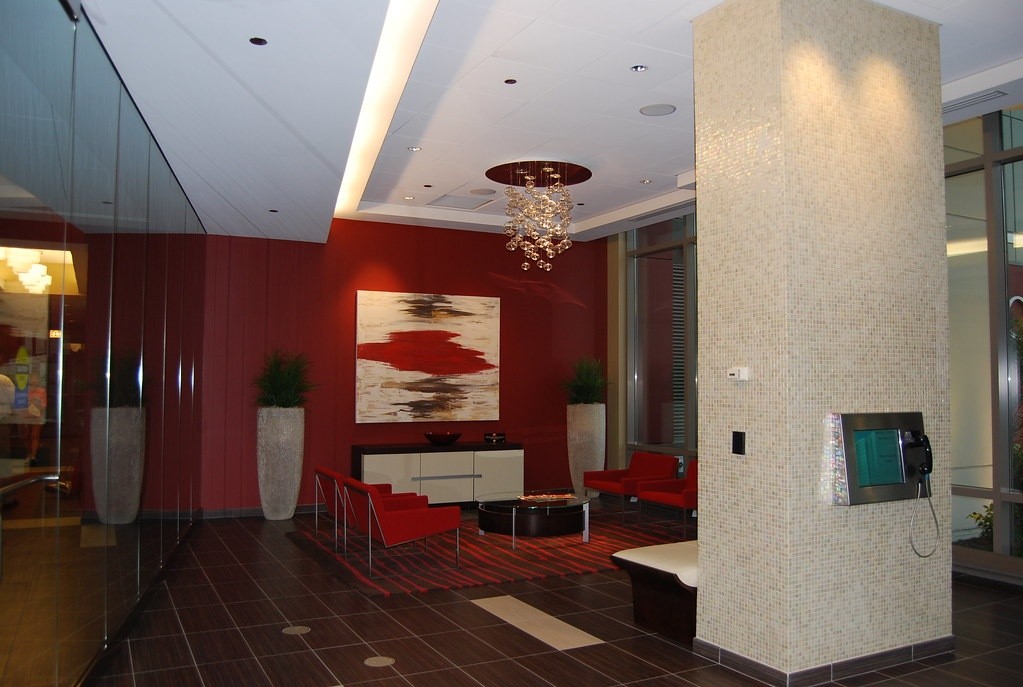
[{"left": 854, "top": 429, "right": 904, "bottom": 487}]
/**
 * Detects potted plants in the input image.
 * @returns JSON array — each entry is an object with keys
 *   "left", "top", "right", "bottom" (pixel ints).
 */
[
  {"left": 566, "top": 355, "right": 608, "bottom": 498},
  {"left": 89, "top": 352, "right": 146, "bottom": 526},
  {"left": 249, "top": 350, "right": 321, "bottom": 519}
]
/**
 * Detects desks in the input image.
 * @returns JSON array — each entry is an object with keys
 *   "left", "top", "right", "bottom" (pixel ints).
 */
[{"left": 475, "top": 487, "right": 600, "bottom": 550}]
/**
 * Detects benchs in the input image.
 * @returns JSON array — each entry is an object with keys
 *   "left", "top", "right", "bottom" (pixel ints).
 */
[{"left": 609, "top": 538, "right": 696, "bottom": 646}]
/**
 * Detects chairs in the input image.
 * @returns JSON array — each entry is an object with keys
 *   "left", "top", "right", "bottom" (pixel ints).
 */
[
  {"left": 342, "top": 476, "right": 461, "bottom": 582},
  {"left": 582, "top": 448, "right": 680, "bottom": 521},
  {"left": 635, "top": 456, "right": 697, "bottom": 539},
  {"left": 313, "top": 463, "right": 417, "bottom": 555}
]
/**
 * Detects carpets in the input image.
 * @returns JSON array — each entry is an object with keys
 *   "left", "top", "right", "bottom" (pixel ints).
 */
[{"left": 285, "top": 492, "right": 697, "bottom": 598}]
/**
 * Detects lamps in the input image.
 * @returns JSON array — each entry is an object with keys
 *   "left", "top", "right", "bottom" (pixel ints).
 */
[
  {"left": 504, "top": 161, "right": 574, "bottom": 271},
  {"left": 6, "top": 248, "right": 53, "bottom": 295}
]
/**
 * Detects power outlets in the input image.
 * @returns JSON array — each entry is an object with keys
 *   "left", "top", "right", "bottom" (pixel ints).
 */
[{"left": 732, "top": 432, "right": 745, "bottom": 455}]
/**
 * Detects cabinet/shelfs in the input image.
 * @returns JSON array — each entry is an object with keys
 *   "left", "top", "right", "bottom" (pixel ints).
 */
[{"left": 351, "top": 444, "right": 526, "bottom": 506}]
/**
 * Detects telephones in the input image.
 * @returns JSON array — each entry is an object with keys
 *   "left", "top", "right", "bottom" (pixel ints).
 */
[{"left": 917, "top": 433, "right": 933, "bottom": 474}]
